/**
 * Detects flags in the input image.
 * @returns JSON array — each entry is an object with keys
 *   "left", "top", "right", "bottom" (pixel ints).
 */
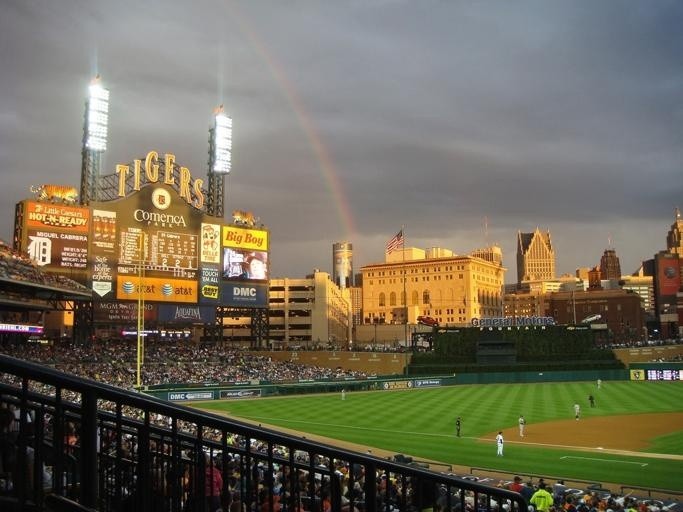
[{"left": 386, "top": 230, "right": 403, "bottom": 255}]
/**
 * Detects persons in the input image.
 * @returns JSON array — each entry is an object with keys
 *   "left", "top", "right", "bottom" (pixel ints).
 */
[
  {"left": 1, "top": 337, "right": 682, "bottom": 512},
  {"left": 246, "top": 252, "right": 267, "bottom": 281}
]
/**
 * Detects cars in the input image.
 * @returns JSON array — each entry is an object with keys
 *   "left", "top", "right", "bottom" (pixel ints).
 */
[
  {"left": 581, "top": 314, "right": 602, "bottom": 324},
  {"left": 416, "top": 314, "right": 439, "bottom": 326}
]
